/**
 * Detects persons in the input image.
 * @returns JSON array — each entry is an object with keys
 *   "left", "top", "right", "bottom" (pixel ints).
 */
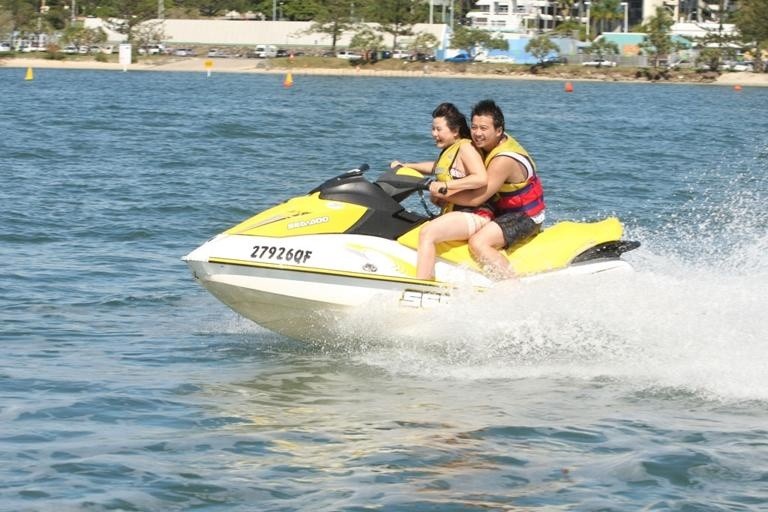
[
  {"left": 429, "top": 100, "right": 546, "bottom": 283},
  {"left": 390, "top": 103, "right": 499, "bottom": 282}
]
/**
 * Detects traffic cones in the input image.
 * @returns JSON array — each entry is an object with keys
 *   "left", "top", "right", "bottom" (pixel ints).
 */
[{"left": 288, "top": 51, "right": 296, "bottom": 61}]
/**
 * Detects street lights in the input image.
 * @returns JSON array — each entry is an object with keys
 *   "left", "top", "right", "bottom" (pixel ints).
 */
[
  {"left": 583, "top": 2, "right": 591, "bottom": 42},
  {"left": 620, "top": 2, "right": 628, "bottom": 32}
]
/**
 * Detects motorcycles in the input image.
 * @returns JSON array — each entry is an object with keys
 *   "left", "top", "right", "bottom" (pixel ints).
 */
[{"left": 177, "top": 161, "right": 645, "bottom": 344}]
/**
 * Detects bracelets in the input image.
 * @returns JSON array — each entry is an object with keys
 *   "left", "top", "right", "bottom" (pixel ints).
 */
[{"left": 443, "top": 180, "right": 448, "bottom": 191}]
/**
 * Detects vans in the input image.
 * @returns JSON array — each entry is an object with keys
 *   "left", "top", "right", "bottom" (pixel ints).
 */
[
  {"left": 137, "top": 43, "right": 173, "bottom": 57},
  {"left": 254, "top": 44, "right": 279, "bottom": 58}
]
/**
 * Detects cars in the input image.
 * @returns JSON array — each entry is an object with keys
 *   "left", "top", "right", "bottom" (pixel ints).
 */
[
  {"left": 483, "top": 55, "right": 512, "bottom": 64},
  {"left": 277, "top": 50, "right": 288, "bottom": 58},
  {"left": 548, "top": 55, "right": 568, "bottom": 66},
  {"left": 399, "top": 52, "right": 436, "bottom": 62},
  {"left": 337, "top": 51, "right": 362, "bottom": 59},
  {"left": 443, "top": 53, "right": 474, "bottom": 63},
  {"left": 667, "top": 57, "right": 768, "bottom": 72},
  {"left": 206, "top": 48, "right": 223, "bottom": 58},
  {"left": 582, "top": 57, "right": 617, "bottom": 67},
  {"left": 0, "top": 42, "right": 115, "bottom": 55},
  {"left": 176, "top": 48, "right": 198, "bottom": 57}
]
